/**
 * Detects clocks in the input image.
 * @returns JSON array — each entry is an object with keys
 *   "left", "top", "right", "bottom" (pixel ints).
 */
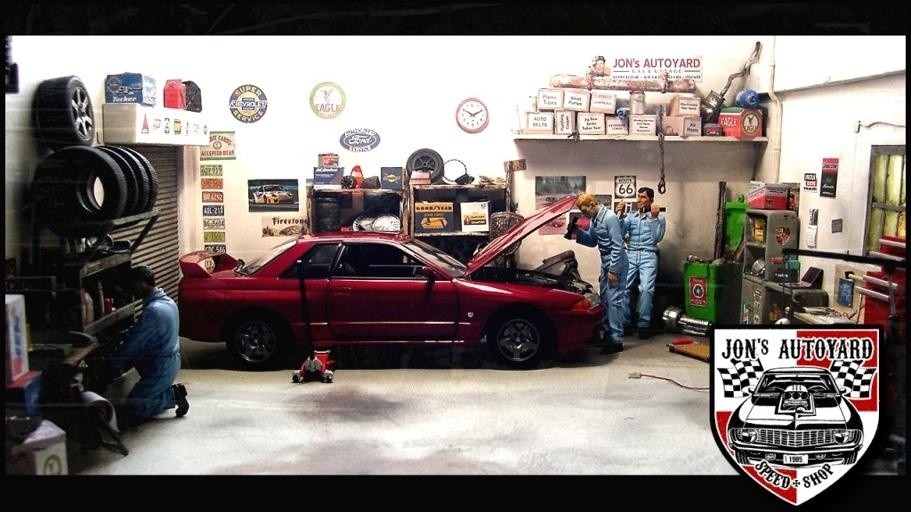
[{"left": 456, "top": 98, "right": 490, "bottom": 133}]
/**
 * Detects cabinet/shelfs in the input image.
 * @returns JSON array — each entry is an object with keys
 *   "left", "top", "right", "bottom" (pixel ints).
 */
[
  {"left": 35, "top": 247, "right": 136, "bottom": 390},
  {"left": 739, "top": 208, "right": 831, "bottom": 324},
  {"left": 306, "top": 181, "right": 511, "bottom": 267}
]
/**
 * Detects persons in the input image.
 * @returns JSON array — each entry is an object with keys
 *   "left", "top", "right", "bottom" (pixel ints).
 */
[
  {"left": 567, "top": 192, "right": 629, "bottom": 355},
  {"left": 96, "top": 264, "right": 190, "bottom": 427},
  {"left": 615, "top": 186, "right": 667, "bottom": 339}
]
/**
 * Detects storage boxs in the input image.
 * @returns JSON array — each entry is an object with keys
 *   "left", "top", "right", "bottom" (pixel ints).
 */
[
  {"left": 525, "top": 86, "right": 763, "bottom": 138},
  {"left": 6, "top": 293, "right": 69, "bottom": 476}
]
